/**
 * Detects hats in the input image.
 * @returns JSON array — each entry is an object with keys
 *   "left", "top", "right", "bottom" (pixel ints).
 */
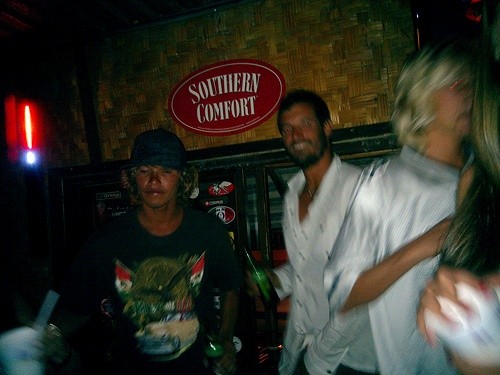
[{"left": 133, "top": 129, "right": 187, "bottom": 171}]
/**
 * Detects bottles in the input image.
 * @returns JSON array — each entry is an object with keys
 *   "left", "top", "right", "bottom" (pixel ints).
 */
[
  {"left": 205, "top": 327, "right": 228, "bottom": 375},
  {"left": 242, "top": 244, "right": 281, "bottom": 308}
]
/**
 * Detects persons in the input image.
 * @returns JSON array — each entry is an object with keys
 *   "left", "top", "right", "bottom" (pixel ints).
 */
[
  {"left": 243, "top": 88, "right": 383, "bottom": 375},
  {"left": 321, "top": 38, "right": 500, "bottom": 375},
  {"left": 85, "top": 125, "right": 258, "bottom": 375}
]
